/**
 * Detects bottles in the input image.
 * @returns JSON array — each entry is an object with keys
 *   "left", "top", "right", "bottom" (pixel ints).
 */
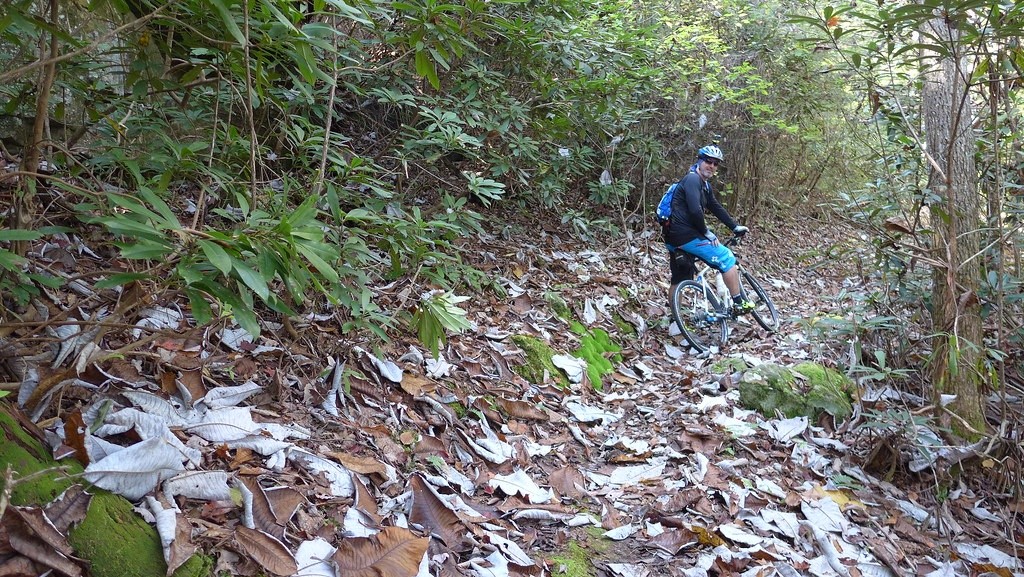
[{"left": 716, "top": 272, "right": 724, "bottom": 297}]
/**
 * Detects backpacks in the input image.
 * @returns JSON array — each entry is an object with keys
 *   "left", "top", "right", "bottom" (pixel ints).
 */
[{"left": 655, "top": 170, "right": 703, "bottom": 227}]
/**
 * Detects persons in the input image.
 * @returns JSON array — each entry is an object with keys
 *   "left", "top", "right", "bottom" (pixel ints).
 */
[{"left": 665, "top": 145, "right": 755, "bottom": 336}]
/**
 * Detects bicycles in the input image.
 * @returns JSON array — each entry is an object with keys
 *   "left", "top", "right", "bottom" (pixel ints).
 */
[{"left": 671, "top": 229, "right": 779, "bottom": 353}]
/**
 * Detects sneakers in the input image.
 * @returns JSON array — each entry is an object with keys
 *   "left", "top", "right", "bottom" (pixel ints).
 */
[
  {"left": 733, "top": 299, "right": 756, "bottom": 315},
  {"left": 672, "top": 306, "right": 690, "bottom": 319}
]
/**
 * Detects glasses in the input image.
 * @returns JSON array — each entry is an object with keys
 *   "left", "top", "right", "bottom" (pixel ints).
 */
[{"left": 705, "top": 158, "right": 718, "bottom": 166}]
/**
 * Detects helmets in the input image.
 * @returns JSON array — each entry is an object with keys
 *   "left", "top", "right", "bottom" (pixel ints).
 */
[{"left": 698, "top": 145, "right": 724, "bottom": 163}]
